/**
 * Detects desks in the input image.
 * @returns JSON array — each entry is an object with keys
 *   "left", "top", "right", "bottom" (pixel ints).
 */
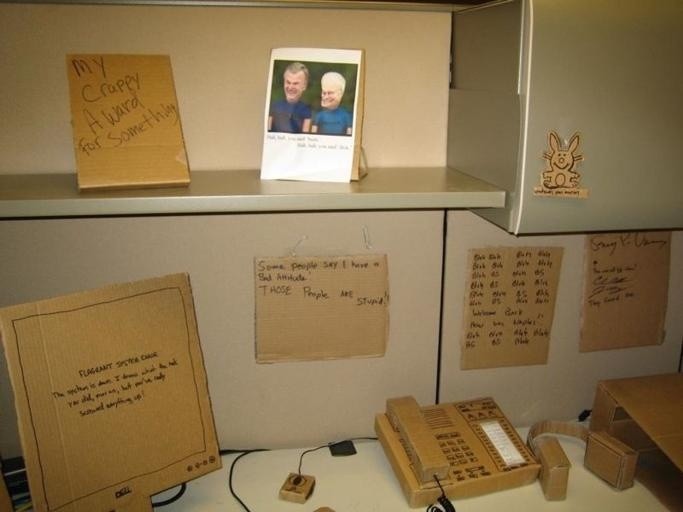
[{"left": 0, "top": 424, "right": 680, "bottom": 511}]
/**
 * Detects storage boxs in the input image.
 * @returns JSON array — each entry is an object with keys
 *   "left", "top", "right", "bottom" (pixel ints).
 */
[{"left": 592, "top": 373, "right": 683, "bottom": 511}]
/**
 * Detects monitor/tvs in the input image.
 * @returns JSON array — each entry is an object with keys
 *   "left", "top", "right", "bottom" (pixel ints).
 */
[{"left": 1, "top": 271, "right": 222, "bottom": 512}]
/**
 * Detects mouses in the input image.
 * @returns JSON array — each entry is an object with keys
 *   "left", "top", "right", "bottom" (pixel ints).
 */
[{"left": 280, "top": 472, "right": 316, "bottom": 504}]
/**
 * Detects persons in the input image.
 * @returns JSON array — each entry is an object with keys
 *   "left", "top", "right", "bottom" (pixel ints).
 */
[
  {"left": 312, "top": 72, "right": 352, "bottom": 135},
  {"left": 267, "top": 63, "right": 311, "bottom": 134}
]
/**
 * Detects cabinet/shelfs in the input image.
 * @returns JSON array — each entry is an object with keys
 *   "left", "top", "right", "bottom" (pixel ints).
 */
[{"left": 448, "top": 0, "right": 681, "bottom": 237}]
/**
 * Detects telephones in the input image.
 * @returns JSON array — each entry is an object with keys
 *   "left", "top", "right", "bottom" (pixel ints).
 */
[{"left": 374, "top": 396, "right": 541, "bottom": 506}]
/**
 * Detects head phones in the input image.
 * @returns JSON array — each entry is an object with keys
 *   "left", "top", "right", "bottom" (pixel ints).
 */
[{"left": 528, "top": 417, "right": 640, "bottom": 502}]
